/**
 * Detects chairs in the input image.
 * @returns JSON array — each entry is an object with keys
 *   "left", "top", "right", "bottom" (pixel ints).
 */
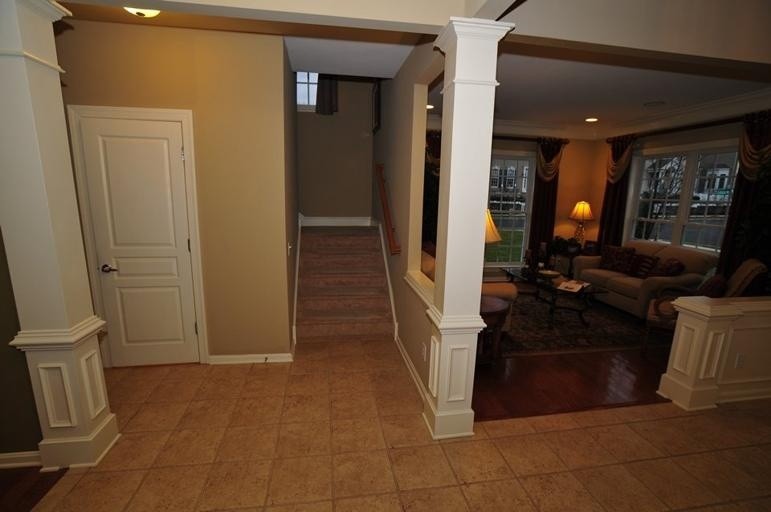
[
  {"left": 422, "top": 250, "right": 517, "bottom": 332},
  {"left": 641, "top": 258, "right": 768, "bottom": 362}
]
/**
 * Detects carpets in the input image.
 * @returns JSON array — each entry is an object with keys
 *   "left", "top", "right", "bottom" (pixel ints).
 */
[
  {"left": 500, "top": 291, "right": 675, "bottom": 356},
  {"left": 0, "top": 464, "right": 68, "bottom": 512}
]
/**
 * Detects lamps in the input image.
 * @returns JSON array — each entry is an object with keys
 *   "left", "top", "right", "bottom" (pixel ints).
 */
[
  {"left": 486, "top": 209, "right": 503, "bottom": 244},
  {"left": 123, "top": 7, "right": 160, "bottom": 17},
  {"left": 567, "top": 200, "right": 593, "bottom": 248}
]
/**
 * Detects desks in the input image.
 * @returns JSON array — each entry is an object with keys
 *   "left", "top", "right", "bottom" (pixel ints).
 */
[{"left": 475, "top": 296, "right": 510, "bottom": 372}]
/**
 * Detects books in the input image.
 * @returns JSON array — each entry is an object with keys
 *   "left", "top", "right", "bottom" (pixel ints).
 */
[{"left": 557, "top": 278, "right": 592, "bottom": 294}]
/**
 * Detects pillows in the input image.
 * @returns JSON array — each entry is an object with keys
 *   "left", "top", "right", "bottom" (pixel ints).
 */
[
  {"left": 596, "top": 244, "right": 686, "bottom": 277},
  {"left": 695, "top": 274, "right": 728, "bottom": 298}
]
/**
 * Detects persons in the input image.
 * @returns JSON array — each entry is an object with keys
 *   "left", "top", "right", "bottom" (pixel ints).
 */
[{"left": 597, "top": 240, "right": 685, "bottom": 278}]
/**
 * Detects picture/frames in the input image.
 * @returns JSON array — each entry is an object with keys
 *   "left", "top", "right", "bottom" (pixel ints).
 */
[
  {"left": 582, "top": 240, "right": 598, "bottom": 256},
  {"left": 370, "top": 84, "right": 381, "bottom": 135}
]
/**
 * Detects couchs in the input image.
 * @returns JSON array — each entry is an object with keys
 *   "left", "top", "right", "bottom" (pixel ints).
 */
[{"left": 572, "top": 239, "right": 720, "bottom": 326}]
